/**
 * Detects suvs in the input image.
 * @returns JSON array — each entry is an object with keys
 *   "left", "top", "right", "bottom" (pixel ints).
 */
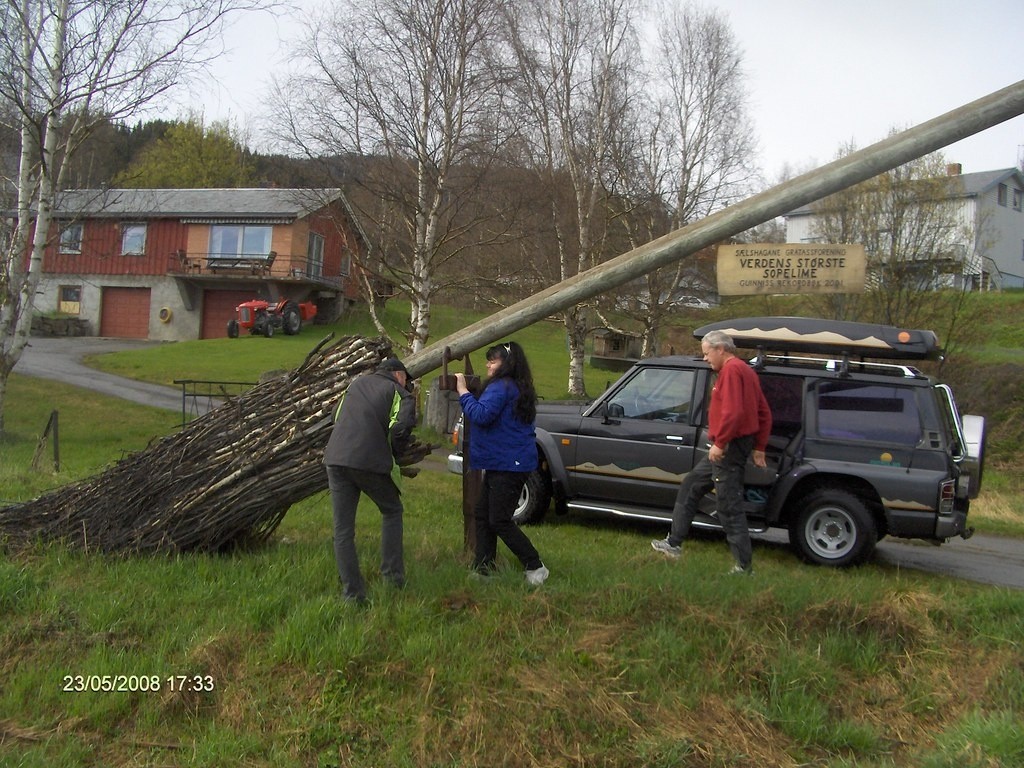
[{"left": 447, "top": 316, "right": 988, "bottom": 576}]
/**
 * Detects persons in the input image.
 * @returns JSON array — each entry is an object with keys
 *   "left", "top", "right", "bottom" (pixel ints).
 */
[
  {"left": 322, "top": 359, "right": 418, "bottom": 612},
  {"left": 651, "top": 330, "right": 772, "bottom": 580},
  {"left": 455, "top": 341, "right": 550, "bottom": 589}
]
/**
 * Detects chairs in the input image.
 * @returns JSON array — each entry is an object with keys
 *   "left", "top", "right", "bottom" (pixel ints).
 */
[
  {"left": 252, "top": 251, "right": 277, "bottom": 275},
  {"left": 177, "top": 248, "right": 201, "bottom": 274}
]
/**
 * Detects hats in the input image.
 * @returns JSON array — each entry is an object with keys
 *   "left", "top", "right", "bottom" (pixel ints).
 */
[{"left": 376, "top": 359, "right": 413, "bottom": 381}]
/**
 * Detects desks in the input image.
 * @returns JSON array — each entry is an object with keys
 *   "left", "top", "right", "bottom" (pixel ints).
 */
[{"left": 202, "top": 257, "right": 265, "bottom": 273}]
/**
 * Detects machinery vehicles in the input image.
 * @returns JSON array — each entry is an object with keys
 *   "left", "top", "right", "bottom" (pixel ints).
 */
[{"left": 226, "top": 296, "right": 317, "bottom": 338}]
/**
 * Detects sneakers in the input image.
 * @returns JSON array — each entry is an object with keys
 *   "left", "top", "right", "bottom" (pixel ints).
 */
[
  {"left": 728, "top": 567, "right": 755, "bottom": 578},
  {"left": 650, "top": 532, "right": 682, "bottom": 559},
  {"left": 522, "top": 560, "right": 549, "bottom": 585},
  {"left": 470, "top": 573, "right": 497, "bottom": 584}
]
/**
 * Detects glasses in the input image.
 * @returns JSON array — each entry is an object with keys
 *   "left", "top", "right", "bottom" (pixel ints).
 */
[{"left": 503, "top": 343, "right": 510, "bottom": 355}]
